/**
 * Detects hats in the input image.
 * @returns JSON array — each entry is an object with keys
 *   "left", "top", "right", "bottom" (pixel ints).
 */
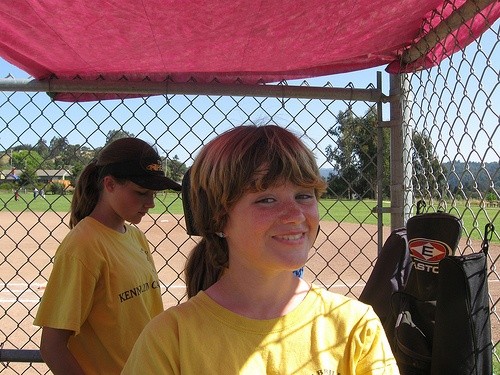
[{"left": 95, "top": 138, "right": 182, "bottom": 192}]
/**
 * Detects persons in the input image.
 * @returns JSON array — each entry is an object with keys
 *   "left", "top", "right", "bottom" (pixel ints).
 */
[
  {"left": 15, "top": 186, "right": 46, "bottom": 200},
  {"left": 120, "top": 125, "right": 401, "bottom": 374},
  {"left": 32, "top": 137, "right": 182, "bottom": 375}
]
